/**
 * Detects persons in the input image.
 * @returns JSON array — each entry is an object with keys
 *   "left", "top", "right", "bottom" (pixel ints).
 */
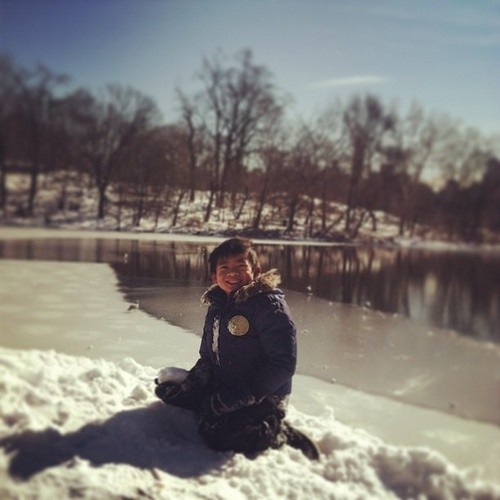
[{"left": 154, "top": 238, "right": 320, "bottom": 461}]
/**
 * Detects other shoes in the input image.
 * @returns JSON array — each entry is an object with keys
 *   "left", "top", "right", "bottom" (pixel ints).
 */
[{"left": 284, "top": 419, "right": 320, "bottom": 460}]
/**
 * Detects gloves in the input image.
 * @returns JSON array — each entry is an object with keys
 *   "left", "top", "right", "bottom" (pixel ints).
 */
[
  {"left": 155, "top": 381, "right": 201, "bottom": 410},
  {"left": 198, "top": 387, "right": 255, "bottom": 424}
]
